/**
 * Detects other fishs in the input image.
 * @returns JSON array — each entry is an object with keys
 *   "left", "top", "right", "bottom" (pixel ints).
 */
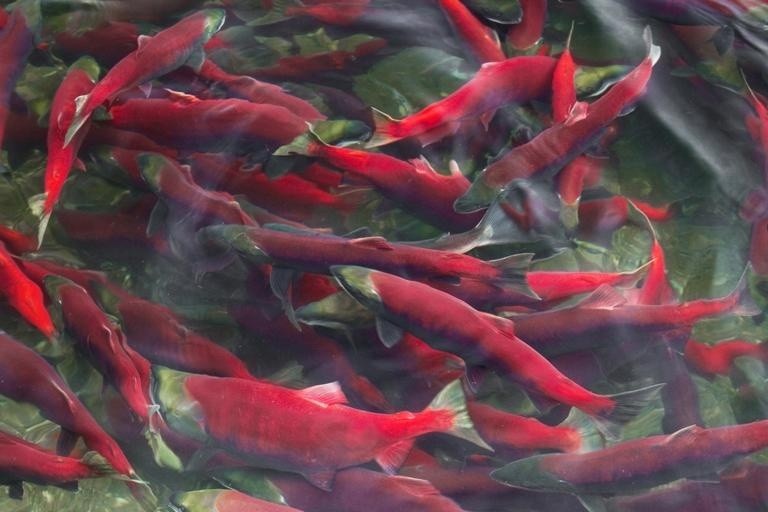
[{"left": 0, "top": 0, "right": 768, "bottom": 512}]
